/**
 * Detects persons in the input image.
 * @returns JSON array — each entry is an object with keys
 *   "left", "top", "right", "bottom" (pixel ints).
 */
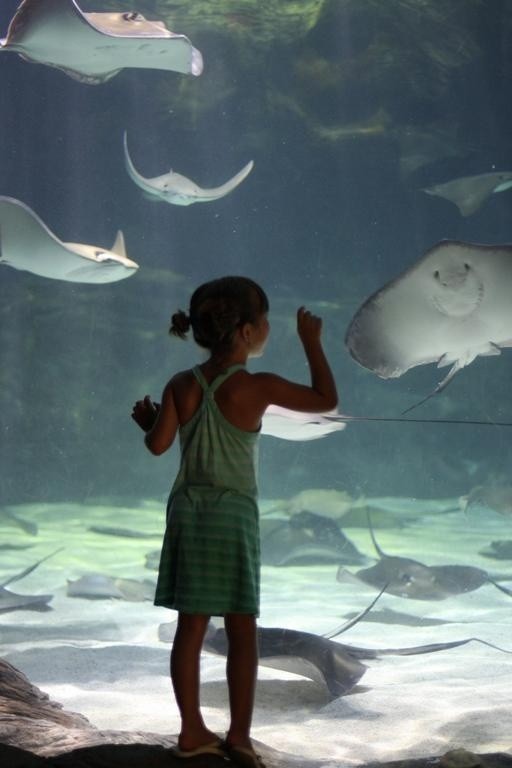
[{"left": 129, "top": 274, "right": 339, "bottom": 767}]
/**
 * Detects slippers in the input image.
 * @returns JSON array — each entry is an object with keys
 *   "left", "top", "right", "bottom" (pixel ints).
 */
[
  {"left": 169, "top": 736, "right": 225, "bottom": 758},
  {"left": 228, "top": 746, "right": 264, "bottom": 767}
]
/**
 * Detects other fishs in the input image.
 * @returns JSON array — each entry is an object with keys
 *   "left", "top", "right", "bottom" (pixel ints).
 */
[
  {"left": 155, "top": 614, "right": 511, "bottom": 696},
  {"left": 0, "top": 473, "right": 509, "bottom": 615}
]
